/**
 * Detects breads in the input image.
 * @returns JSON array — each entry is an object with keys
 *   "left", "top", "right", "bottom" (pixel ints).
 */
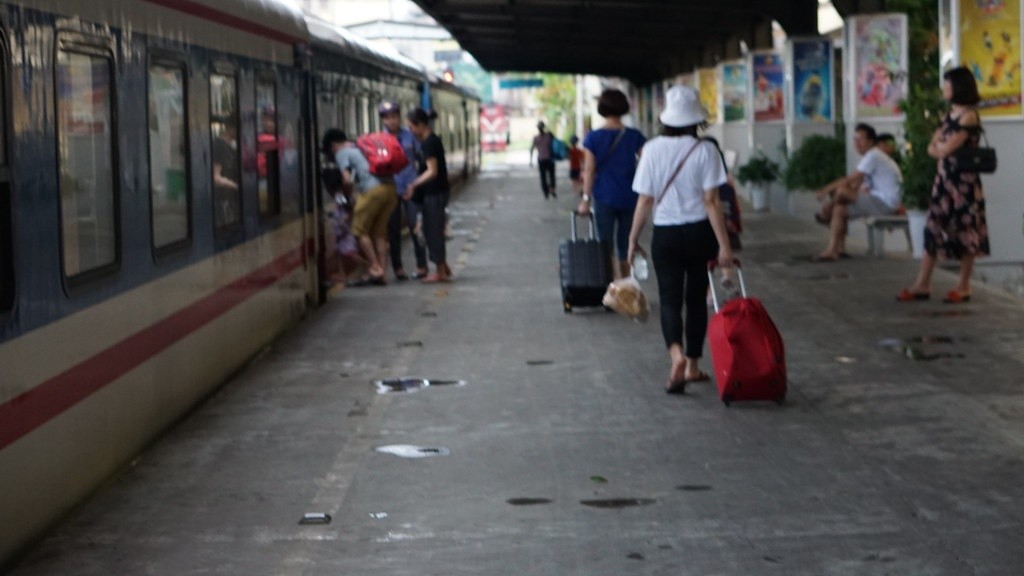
[{"left": 601, "top": 282, "right": 648, "bottom": 322}]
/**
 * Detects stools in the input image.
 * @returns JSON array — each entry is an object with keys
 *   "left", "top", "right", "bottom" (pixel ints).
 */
[{"left": 863, "top": 215, "right": 914, "bottom": 260}]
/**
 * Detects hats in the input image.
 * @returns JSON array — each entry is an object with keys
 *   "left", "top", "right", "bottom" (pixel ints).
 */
[{"left": 659, "top": 85, "right": 708, "bottom": 127}]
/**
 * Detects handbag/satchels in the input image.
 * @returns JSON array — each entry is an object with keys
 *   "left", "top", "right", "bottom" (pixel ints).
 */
[
  {"left": 943, "top": 109, "right": 998, "bottom": 177},
  {"left": 601, "top": 263, "right": 651, "bottom": 324}
]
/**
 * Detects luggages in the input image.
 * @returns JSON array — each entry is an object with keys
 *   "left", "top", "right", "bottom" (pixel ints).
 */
[
  {"left": 706, "top": 256, "right": 788, "bottom": 407},
  {"left": 558, "top": 209, "right": 609, "bottom": 313}
]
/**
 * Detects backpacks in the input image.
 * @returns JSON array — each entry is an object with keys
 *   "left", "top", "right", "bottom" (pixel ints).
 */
[{"left": 354, "top": 131, "right": 408, "bottom": 177}]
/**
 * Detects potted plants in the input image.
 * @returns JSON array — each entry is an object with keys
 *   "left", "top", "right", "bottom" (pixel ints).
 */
[
  {"left": 786, "top": 135, "right": 849, "bottom": 224},
  {"left": 739, "top": 156, "right": 780, "bottom": 212},
  {"left": 900, "top": 82, "right": 951, "bottom": 258}
]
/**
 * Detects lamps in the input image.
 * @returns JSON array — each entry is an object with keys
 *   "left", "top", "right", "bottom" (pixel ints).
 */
[{"left": 769, "top": 0, "right": 846, "bottom": 45}]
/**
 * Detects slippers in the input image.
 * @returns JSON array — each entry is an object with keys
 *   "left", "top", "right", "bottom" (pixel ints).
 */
[
  {"left": 664, "top": 377, "right": 687, "bottom": 394},
  {"left": 896, "top": 288, "right": 931, "bottom": 302},
  {"left": 944, "top": 290, "right": 971, "bottom": 302},
  {"left": 683, "top": 369, "right": 710, "bottom": 384}
]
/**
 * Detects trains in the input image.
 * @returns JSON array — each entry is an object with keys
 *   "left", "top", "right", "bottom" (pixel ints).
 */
[{"left": 0, "top": 1, "right": 482, "bottom": 576}]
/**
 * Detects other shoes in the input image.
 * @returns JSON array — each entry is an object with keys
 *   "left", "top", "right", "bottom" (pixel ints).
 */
[
  {"left": 346, "top": 271, "right": 383, "bottom": 287},
  {"left": 412, "top": 268, "right": 427, "bottom": 277},
  {"left": 396, "top": 271, "right": 408, "bottom": 281},
  {"left": 422, "top": 274, "right": 446, "bottom": 283}
]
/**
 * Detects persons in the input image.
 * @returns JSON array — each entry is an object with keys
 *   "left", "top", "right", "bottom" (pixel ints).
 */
[
  {"left": 577, "top": 88, "right": 647, "bottom": 314},
  {"left": 568, "top": 134, "right": 585, "bottom": 196},
  {"left": 530, "top": 120, "right": 558, "bottom": 200},
  {"left": 627, "top": 85, "right": 733, "bottom": 394},
  {"left": 699, "top": 136, "right": 740, "bottom": 309},
  {"left": 808, "top": 123, "right": 906, "bottom": 263},
  {"left": 208, "top": 105, "right": 299, "bottom": 228},
  {"left": 895, "top": 66, "right": 990, "bottom": 303},
  {"left": 323, "top": 98, "right": 453, "bottom": 287}
]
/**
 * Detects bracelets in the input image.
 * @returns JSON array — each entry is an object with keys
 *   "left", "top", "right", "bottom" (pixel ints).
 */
[{"left": 583, "top": 194, "right": 591, "bottom": 201}]
